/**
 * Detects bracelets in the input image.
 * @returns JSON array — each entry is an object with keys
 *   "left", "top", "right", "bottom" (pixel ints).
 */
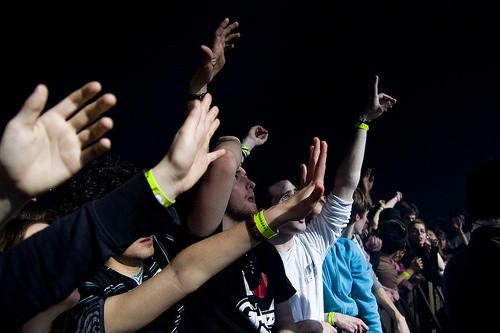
[
  {"left": 328, "top": 311, "right": 335, "bottom": 326},
  {"left": 355, "top": 122, "right": 369, "bottom": 131},
  {"left": 358, "top": 115, "right": 374, "bottom": 125},
  {"left": 143, "top": 168, "right": 176, "bottom": 209},
  {"left": 402, "top": 271, "right": 411, "bottom": 280},
  {"left": 253, "top": 208, "right": 280, "bottom": 240},
  {"left": 247, "top": 213, "right": 263, "bottom": 243},
  {"left": 187, "top": 91, "right": 207, "bottom": 102},
  {"left": 238, "top": 143, "right": 251, "bottom": 158}
]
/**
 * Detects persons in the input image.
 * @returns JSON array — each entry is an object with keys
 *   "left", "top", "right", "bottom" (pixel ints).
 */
[{"left": 0, "top": 18, "right": 500, "bottom": 333}]
